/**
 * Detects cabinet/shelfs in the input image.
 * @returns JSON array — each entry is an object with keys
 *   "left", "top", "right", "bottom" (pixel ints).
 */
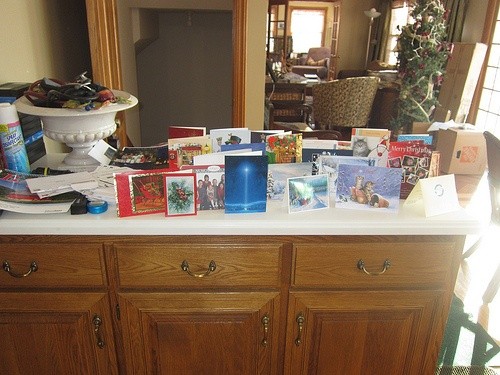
[{"left": 0, "top": 234, "right": 466, "bottom": 375}]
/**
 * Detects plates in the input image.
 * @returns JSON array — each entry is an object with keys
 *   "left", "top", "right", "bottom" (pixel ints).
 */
[{"left": 109, "top": 158, "right": 157, "bottom": 170}]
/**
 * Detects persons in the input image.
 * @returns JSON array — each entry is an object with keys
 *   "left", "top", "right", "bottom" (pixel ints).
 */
[
  {"left": 197, "top": 174, "right": 225, "bottom": 209},
  {"left": 391, "top": 157, "right": 427, "bottom": 183},
  {"left": 122, "top": 152, "right": 156, "bottom": 163},
  {"left": 215, "top": 136, "right": 223, "bottom": 152}
]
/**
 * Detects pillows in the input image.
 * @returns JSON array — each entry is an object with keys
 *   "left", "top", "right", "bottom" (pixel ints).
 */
[{"left": 366, "top": 68, "right": 398, "bottom": 82}]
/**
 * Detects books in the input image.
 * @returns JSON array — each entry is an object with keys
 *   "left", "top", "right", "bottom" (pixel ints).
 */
[{"left": 0, "top": 168, "right": 78, "bottom": 213}]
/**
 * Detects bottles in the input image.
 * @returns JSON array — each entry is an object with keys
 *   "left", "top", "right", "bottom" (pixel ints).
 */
[{"left": 0, "top": 103, "right": 30, "bottom": 174}]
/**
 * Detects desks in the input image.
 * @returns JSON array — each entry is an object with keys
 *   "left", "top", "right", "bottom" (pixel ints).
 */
[{"left": 265, "top": 82, "right": 308, "bottom": 122}]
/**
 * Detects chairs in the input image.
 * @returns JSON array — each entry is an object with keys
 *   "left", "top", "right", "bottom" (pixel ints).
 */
[
  {"left": 265, "top": 97, "right": 342, "bottom": 139},
  {"left": 463, "top": 129, "right": 500, "bottom": 303},
  {"left": 311, "top": 60, "right": 397, "bottom": 132}
]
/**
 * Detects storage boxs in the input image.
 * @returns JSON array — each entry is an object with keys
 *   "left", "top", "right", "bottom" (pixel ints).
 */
[{"left": 412, "top": 42, "right": 489, "bottom": 175}]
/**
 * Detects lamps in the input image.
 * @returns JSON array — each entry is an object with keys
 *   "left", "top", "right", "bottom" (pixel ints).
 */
[{"left": 364, "top": 7, "right": 382, "bottom": 69}]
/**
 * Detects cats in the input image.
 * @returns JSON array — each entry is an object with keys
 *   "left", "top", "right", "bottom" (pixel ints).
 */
[{"left": 353, "top": 137, "right": 372, "bottom": 157}]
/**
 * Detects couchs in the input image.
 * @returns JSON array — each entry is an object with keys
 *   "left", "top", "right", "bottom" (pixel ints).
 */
[{"left": 292, "top": 46, "right": 331, "bottom": 78}]
